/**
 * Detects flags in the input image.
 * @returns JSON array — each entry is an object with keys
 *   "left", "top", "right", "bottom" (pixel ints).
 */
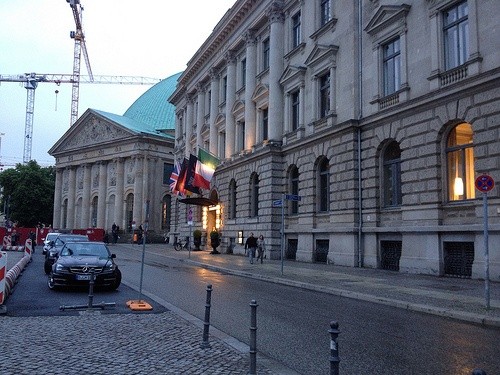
[
  {"left": 169, "top": 171, "right": 182, "bottom": 197},
  {"left": 192, "top": 147, "right": 220, "bottom": 190},
  {"left": 184, "top": 154, "right": 202, "bottom": 195},
  {"left": 174, "top": 157, "right": 193, "bottom": 196}
]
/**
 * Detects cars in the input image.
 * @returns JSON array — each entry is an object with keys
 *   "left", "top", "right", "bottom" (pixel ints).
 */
[
  {"left": 42, "top": 233, "right": 91, "bottom": 274},
  {"left": 47, "top": 242, "right": 122, "bottom": 292}
]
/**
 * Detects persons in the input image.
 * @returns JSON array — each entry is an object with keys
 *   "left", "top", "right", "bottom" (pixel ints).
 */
[
  {"left": 256, "top": 235, "right": 266, "bottom": 264},
  {"left": 245, "top": 232, "right": 257, "bottom": 264},
  {"left": 27, "top": 230, "right": 36, "bottom": 253},
  {"left": 112, "top": 222, "right": 120, "bottom": 243},
  {"left": 137, "top": 224, "right": 144, "bottom": 245},
  {"left": 11, "top": 230, "right": 21, "bottom": 246}
]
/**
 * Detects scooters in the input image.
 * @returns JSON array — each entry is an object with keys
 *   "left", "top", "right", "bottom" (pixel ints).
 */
[{"left": 174, "top": 239, "right": 192, "bottom": 251}]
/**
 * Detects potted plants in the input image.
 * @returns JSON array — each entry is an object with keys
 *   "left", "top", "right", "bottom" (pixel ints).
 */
[
  {"left": 209, "top": 230, "right": 221, "bottom": 254},
  {"left": 191, "top": 230, "right": 203, "bottom": 251}
]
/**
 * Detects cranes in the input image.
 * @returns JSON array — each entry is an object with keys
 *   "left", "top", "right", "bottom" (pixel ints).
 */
[
  {"left": 66, "top": 0, "right": 94, "bottom": 128},
  {"left": 0, "top": 72, "right": 164, "bottom": 165}
]
